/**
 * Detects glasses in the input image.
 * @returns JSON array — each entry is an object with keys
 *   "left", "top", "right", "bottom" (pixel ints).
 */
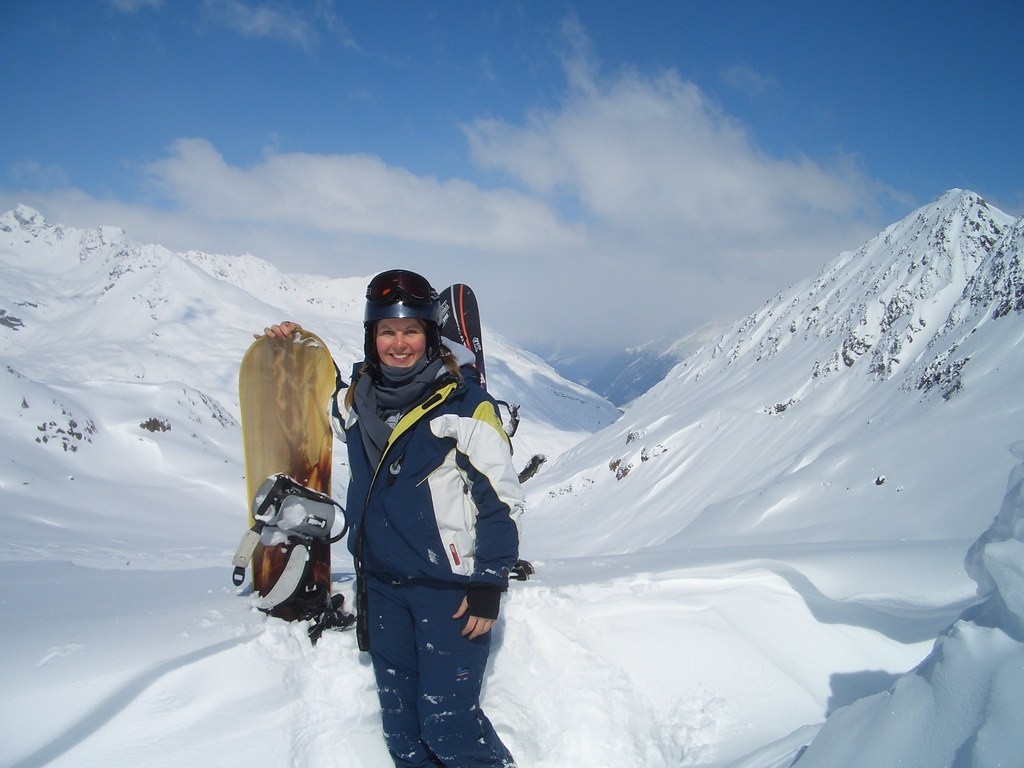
[{"left": 367, "top": 269, "right": 439, "bottom": 307}]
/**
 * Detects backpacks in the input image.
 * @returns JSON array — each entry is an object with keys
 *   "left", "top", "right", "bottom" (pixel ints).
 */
[{"left": 343, "top": 282, "right": 516, "bottom": 457}]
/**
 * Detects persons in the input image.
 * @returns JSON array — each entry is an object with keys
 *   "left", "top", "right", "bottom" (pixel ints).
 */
[{"left": 252, "top": 269, "right": 518, "bottom": 768}]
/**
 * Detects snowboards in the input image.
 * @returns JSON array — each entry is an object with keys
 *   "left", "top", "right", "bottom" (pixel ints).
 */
[
  {"left": 435, "top": 283, "right": 488, "bottom": 395},
  {"left": 237, "top": 329, "right": 337, "bottom": 621}
]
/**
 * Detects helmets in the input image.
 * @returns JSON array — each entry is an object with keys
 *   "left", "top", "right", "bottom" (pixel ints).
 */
[{"left": 363, "top": 270, "right": 442, "bottom": 327}]
